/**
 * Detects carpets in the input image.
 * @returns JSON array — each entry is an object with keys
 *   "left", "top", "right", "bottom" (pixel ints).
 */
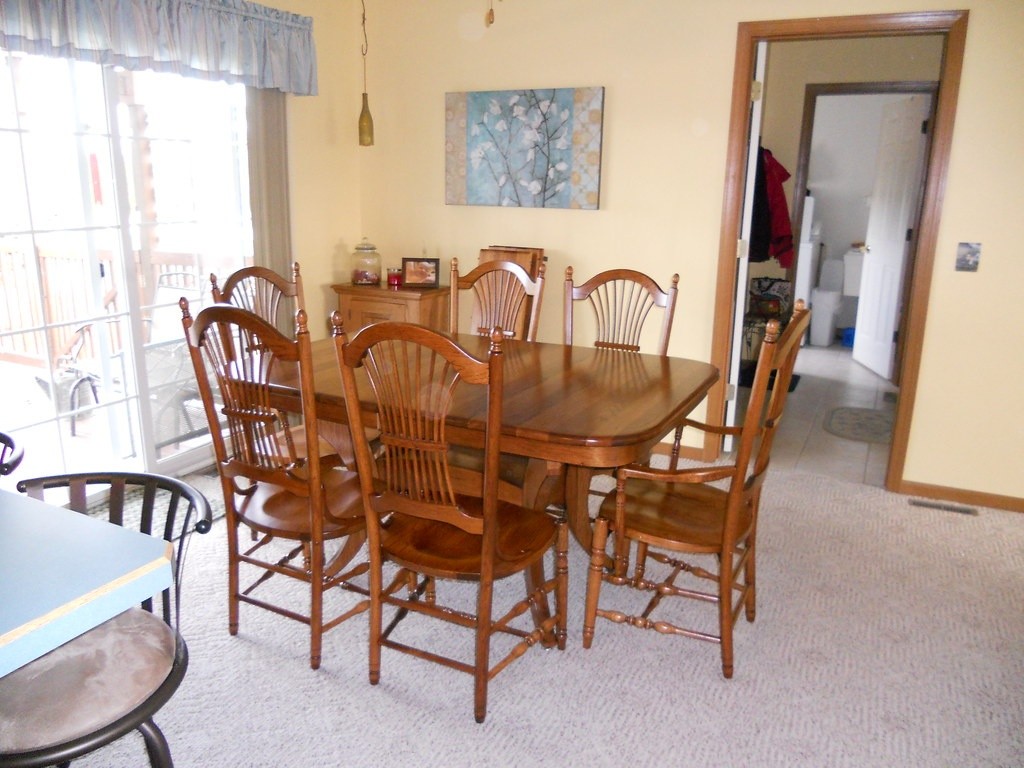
[{"left": 822, "top": 406, "right": 893, "bottom": 445}]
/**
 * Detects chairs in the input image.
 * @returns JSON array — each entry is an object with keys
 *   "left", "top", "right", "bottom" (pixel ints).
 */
[
  {"left": 582, "top": 298, "right": 812, "bottom": 680},
  {"left": 546, "top": 266, "right": 680, "bottom": 536},
  {"left": 209, "top": 262, "right": 382, "bottom": 541},
  {"left": 330, "top": 311, "right": 570, "bottom": 724},
  {"left": 0, "top": 471, "right": 213, "bottom": 768},
  {"left": 179, "top": 296, "right": 418, "bottom": 670},
  {"left": 450, "top": 256, "right": 547, "bottom": 342},
  {"left": 112, "top": 271, "right": 204, "bottom": 449}
]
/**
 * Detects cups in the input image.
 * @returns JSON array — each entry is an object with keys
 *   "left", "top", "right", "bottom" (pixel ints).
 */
[{"left": 387, "top": 267, "right": 402, "bottom": 285}]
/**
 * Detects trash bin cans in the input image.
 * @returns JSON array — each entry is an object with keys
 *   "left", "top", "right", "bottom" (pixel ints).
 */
[{"left": 808, "top": 259, "right": 844, "bottom": 347}]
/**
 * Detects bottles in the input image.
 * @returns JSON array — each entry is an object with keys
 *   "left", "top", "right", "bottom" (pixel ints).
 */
[{"left": 351, "top": 237, "right": 381, "bottom": 285}]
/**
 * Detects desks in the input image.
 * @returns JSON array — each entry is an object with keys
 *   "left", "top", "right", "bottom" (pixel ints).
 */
[
  {"left": 215, "top": 329, "right": 719, "bottom": 573},
  {"left": 0, "top": 487, "right": 177, "bottom": 679}
]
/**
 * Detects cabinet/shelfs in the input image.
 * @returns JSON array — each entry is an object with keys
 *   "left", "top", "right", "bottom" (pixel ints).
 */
[{"left": 330, "top": 280, "right": 451, "bottom": 333}]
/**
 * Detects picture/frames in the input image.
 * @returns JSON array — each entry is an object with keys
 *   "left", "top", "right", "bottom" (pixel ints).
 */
[{"left": 402, "top": 257, "right": 439, "bottom": 289}]
